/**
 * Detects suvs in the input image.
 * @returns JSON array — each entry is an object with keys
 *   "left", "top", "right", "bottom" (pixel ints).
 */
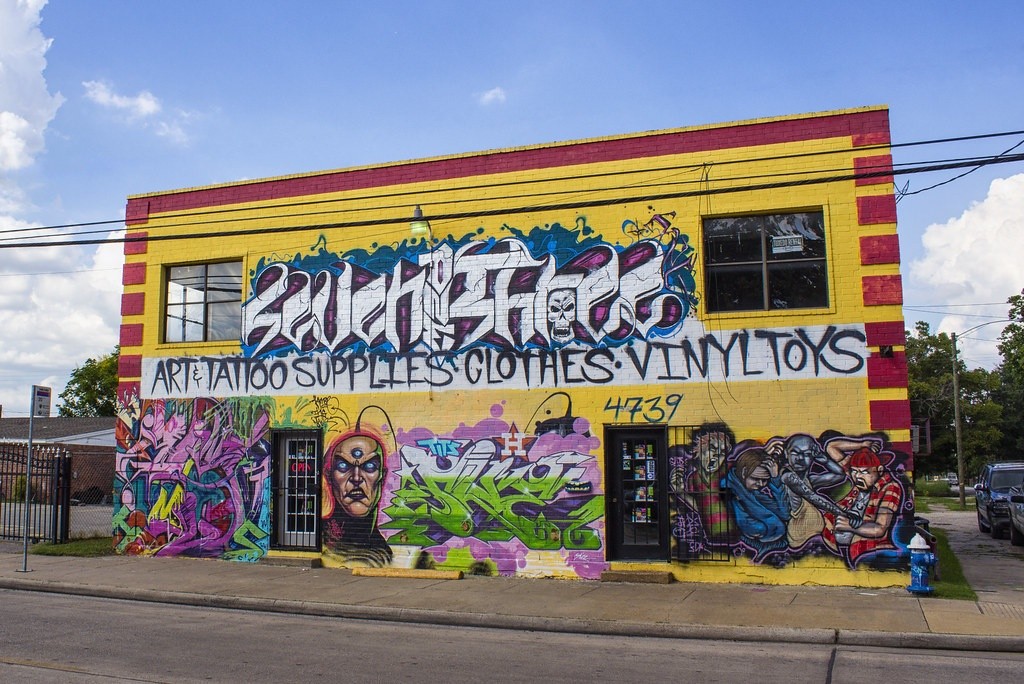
[{"left": 974, "top": 462, "right": 1024, "bottom": 540}]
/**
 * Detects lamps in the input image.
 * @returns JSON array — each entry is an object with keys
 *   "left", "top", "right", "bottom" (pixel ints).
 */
[{"left": 410, "top": 204, "right": 433, "bottom": 267}]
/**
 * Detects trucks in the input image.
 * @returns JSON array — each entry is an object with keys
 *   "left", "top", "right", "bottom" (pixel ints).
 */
[{"left": 947, "top": 472, "right": 958, "bottom": 486}]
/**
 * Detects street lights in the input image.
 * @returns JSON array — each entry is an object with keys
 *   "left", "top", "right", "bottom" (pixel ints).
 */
[{"left": 951, "top": 318, "right": 1024, "bottom": 507}]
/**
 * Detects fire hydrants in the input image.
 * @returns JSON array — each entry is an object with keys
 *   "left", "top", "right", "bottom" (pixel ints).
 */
[{"left": 907, "top": 533, "right": 940, "bottom": 597}]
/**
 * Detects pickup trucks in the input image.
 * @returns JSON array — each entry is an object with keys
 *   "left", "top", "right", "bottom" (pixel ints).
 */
[{"left": 1006, "top": 477, "right": 1024, "bottom": 545}]
